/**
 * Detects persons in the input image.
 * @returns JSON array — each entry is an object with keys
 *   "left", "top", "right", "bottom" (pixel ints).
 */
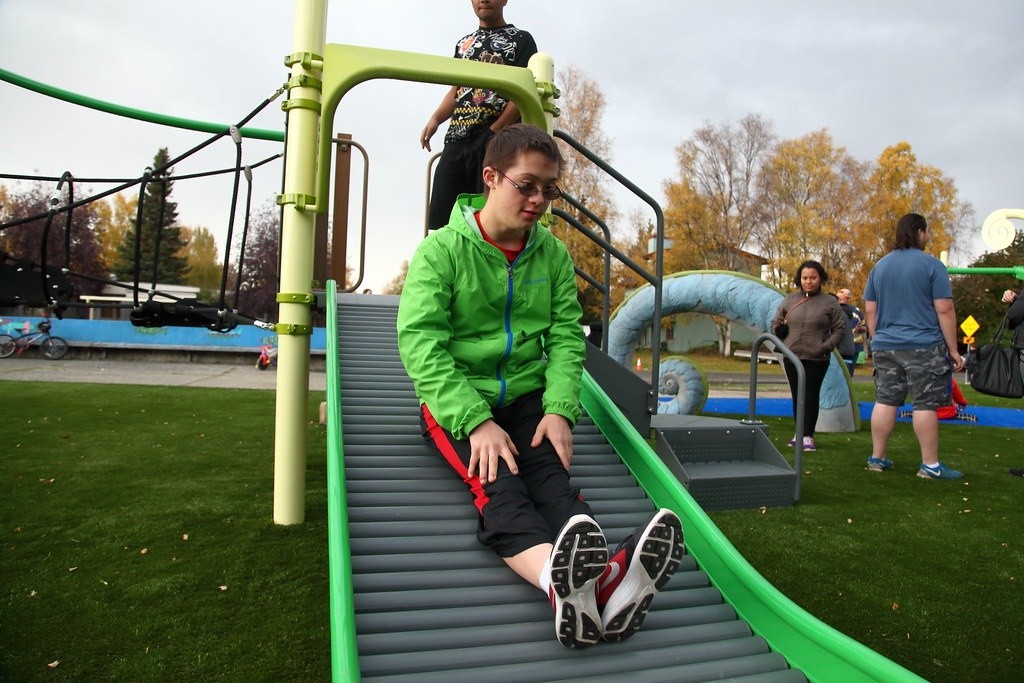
[
  {"left": 1001, "top": 288, "right": 1024, "bottom": 478},
  {"left": 363, "top": 289, "right": 372, "bottom": 294},
  {"left": 771, "top": 261, "right": 846, "bottom": 451},
  {"left": 420, "top": 0, "right": 537, "bottom": 231},
  {"left": 900, "top": 377, "right": 978, "bottom": 422},
  {"left": 861, "top": 214, "right": 964, "bottom": 479},
  {"left": 834, "top": 288, "right": 866, "bottom": 376},
  {"left": 396, "top": 123, "right": 685, "bottom": 649}
]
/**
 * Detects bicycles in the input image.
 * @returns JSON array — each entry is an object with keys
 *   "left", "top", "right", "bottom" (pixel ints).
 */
[{"left": 0, "top": 315, "right": 68, "bottom": 360}]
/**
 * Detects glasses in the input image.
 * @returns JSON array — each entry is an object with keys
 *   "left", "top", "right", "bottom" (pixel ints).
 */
[{"left": 493, "top": 169, "right": 562, "bottom": 200}]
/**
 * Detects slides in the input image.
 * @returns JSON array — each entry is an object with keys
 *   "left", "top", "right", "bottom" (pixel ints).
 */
[{"left": 325, "top": 278, "right": 931, "bottom": 683}]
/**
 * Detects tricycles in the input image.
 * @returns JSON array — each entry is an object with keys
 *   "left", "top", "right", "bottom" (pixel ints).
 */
[{"left": 255, "top": 336, "right": 277, "bottom": 369}]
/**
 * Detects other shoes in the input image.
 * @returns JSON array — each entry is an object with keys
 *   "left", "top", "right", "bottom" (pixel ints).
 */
[{"left": 1009, "top": 468, "right": 1024, "bottom": 478}]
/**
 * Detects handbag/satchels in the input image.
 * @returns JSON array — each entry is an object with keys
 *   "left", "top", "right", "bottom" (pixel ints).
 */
[
  {"left": 967, "top": 308, "right": 1024, "bottom": 398},
  {"left": 773, "top": 324, "right": 789, "bottom": 353}
]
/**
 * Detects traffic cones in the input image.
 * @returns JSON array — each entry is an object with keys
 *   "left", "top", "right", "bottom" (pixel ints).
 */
[{"left": 634, "top": 357, "right": 643, "bottom": 371}]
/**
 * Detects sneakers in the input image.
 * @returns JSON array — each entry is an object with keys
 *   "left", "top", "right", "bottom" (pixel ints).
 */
[
  {"left": 867, "top": 455, "right": 894, "bottom": 471},
  {"left": 802, "top": 436, "right": 816, "bottom": 452},
  {"left": 547, "top": 514, "right": 609, "bottom": 649},
  {"left": 596, "top": 508, "right": 684, "bottom": 642},
  {"left": 787, "top": 434, "right": 796, "bottom": 447},
  {"left": 916, "top": 462, "right": 965, "bottom": 479}
]
